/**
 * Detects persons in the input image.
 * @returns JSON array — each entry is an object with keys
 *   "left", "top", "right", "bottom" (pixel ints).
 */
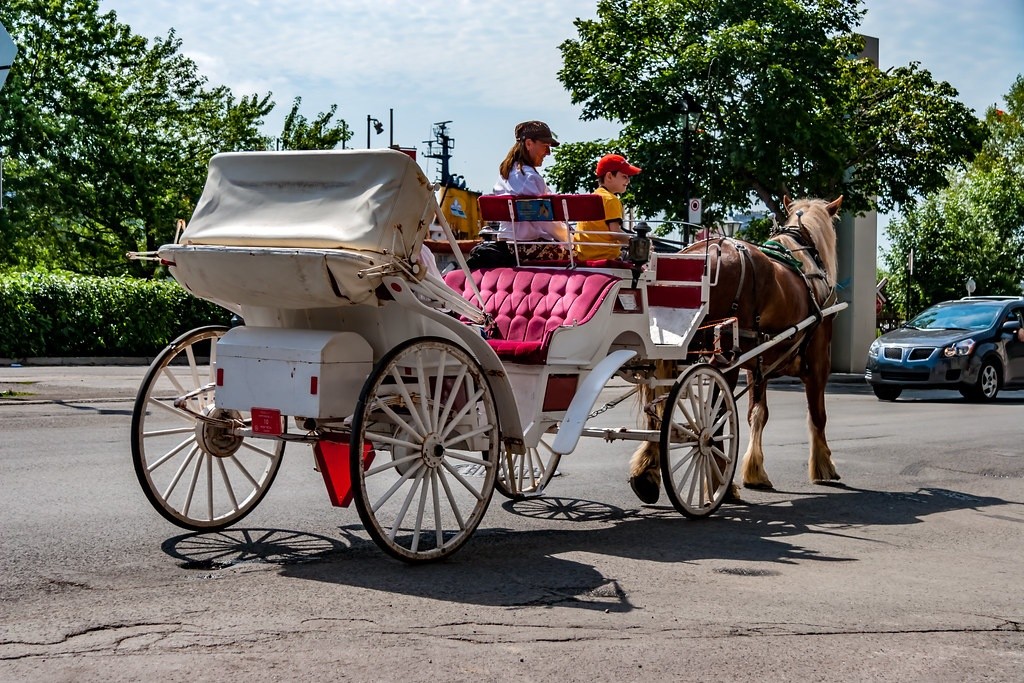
[
  {"left": 576, "top": 154, "right": 652, "bottom": 264},
  {"left": 493, "top": 121, "right": 578, "bottom": 259}
]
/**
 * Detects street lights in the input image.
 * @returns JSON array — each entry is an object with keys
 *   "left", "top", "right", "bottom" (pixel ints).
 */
[{"left": 367, "top": 115, "right": 384, "bottom": 149}]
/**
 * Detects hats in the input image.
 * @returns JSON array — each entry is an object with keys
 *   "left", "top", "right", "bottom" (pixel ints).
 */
[
  {"left": 596, "top": 154, "right": 642, "bottom": 177},
  {"left": 514, "top": 120, "right": 560, "bottom": 147}
]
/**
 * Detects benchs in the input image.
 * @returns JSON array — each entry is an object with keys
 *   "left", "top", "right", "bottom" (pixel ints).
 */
[
  {"left": 478, "top": 194, "right": 638, "bottom": 269},
  {"left": 446, "top": 268, "right": 622, "bottom": 365}
]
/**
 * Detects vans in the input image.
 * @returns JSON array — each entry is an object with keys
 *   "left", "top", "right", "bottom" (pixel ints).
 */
[{"left": 864, "top": 294, "right": 1024, "bottom": 404}]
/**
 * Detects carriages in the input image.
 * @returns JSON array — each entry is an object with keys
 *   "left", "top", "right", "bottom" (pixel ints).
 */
[{"left": 126, "top": 146, "right": 853, "bottom": 562}]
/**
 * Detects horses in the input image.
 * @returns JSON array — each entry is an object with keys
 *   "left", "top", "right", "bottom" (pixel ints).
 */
[{"left": 627, "top": 192, "right": 843, "bottom": 504}]
